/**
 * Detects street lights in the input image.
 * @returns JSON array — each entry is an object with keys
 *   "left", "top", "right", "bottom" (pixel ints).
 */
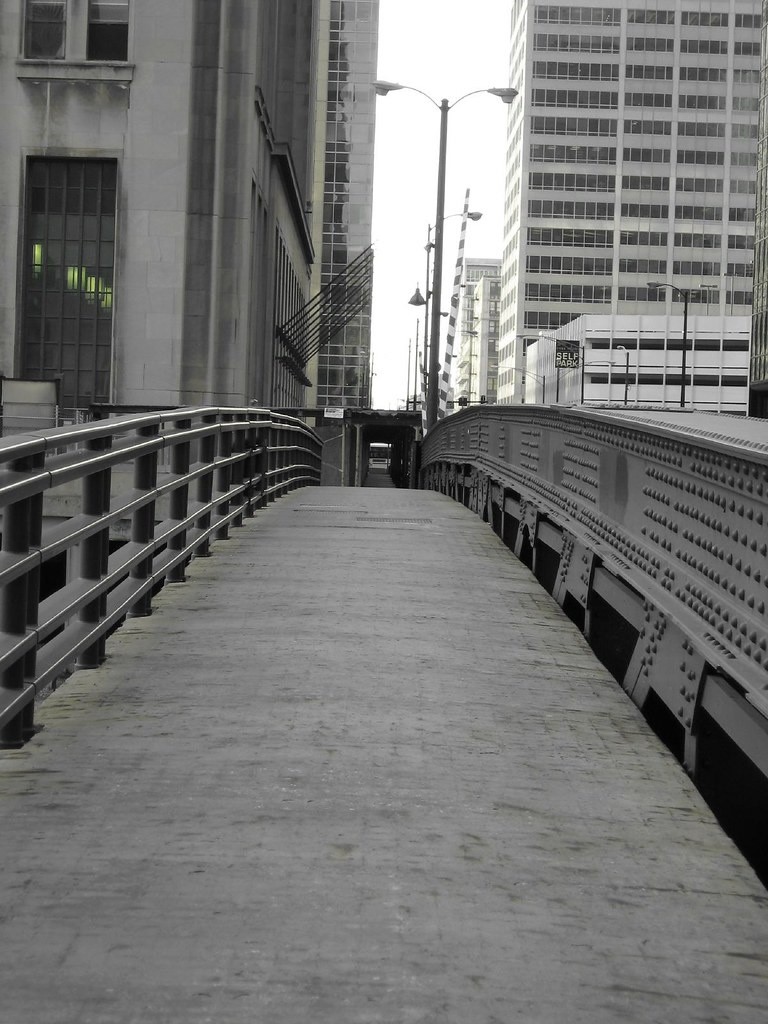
[
  {"left": 371, "top": 79, "right": 520, "bottom": 434},
  {"left": 518, "top": 334, "right": 584, "bottom": 406},
  {"left": 422, "top": 211, "right": 483, "bottom": 404},
  {"left": 647, "top": 281, "right": 687, "bottom": 408},
  {"left": 490, "top": 365, "right": 546, "bottom": 405}
]
